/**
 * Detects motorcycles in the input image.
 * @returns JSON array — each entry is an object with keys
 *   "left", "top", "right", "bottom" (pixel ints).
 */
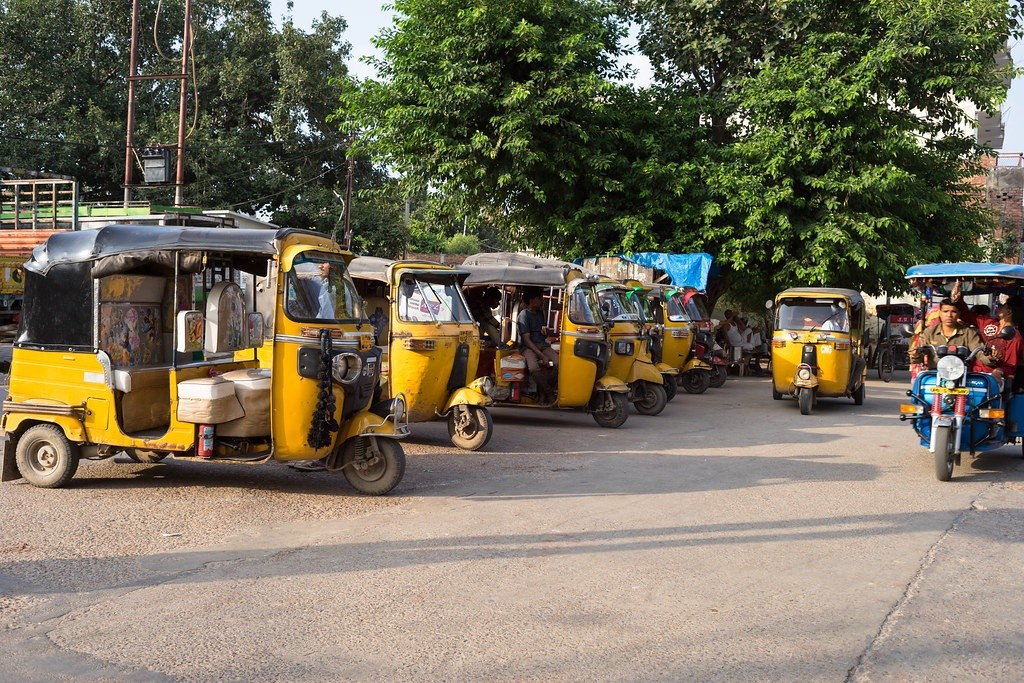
[
  {"left": 898, "top": 263, "right": 1024, "bottom": 482},
  {"left": 765, "top": 286, "right": 869, "bottom": 415},
  {"left": 0, "top": 225, "right": 412, "bottom": 497},
  {"left": 234, "top": 256, "right": 492, "bottom": 451},
  {"left": 453, "top": 251, "right": 727, "bottom": 428}
]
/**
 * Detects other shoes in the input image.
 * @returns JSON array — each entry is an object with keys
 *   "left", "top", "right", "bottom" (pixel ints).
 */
[
  {"left": 286, "top": 459, "right": 326, "bottom": 471},
  {"left": 544, "top": 388, "right": 554, "bottom": 405}
]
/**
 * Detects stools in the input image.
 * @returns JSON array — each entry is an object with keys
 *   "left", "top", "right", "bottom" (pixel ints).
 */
[
  {"left": 216, "top": 368, "right": 271, "bottom": 438},
  {"left": 500, "top": 354, "right": 527, "bottom": 381}
]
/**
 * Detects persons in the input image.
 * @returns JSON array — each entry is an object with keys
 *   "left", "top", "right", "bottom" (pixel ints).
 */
[
  {"left": 907, "top": 299, "right": 1003, "bottom": 373},
  {"left": 283, "top": 246, "right": 353, "bottom": 470},
  {"left": 722, "top": 309, "right": 770, "bottom": 371},
  {"left": 949, "top": 279, "right": 1024, "bottom": 400},
  {"left": 472, "top": 286, "right": 501, "bottom": 345},
  {"left": 821, "top": 306, "right": 866, "bottom": 394},
  {"left": 515, "top": 285, "right": 560, "bottom": 403}
]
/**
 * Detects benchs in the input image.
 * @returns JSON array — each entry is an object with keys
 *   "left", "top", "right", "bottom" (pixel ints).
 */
[{"left": 114, "top": 363, "right": 171, "bottom": 434}]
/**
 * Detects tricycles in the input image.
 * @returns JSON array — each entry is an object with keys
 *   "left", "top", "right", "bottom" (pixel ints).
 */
[{"left": 876, "top": 303, "right": 919, "bottom": 383}]
